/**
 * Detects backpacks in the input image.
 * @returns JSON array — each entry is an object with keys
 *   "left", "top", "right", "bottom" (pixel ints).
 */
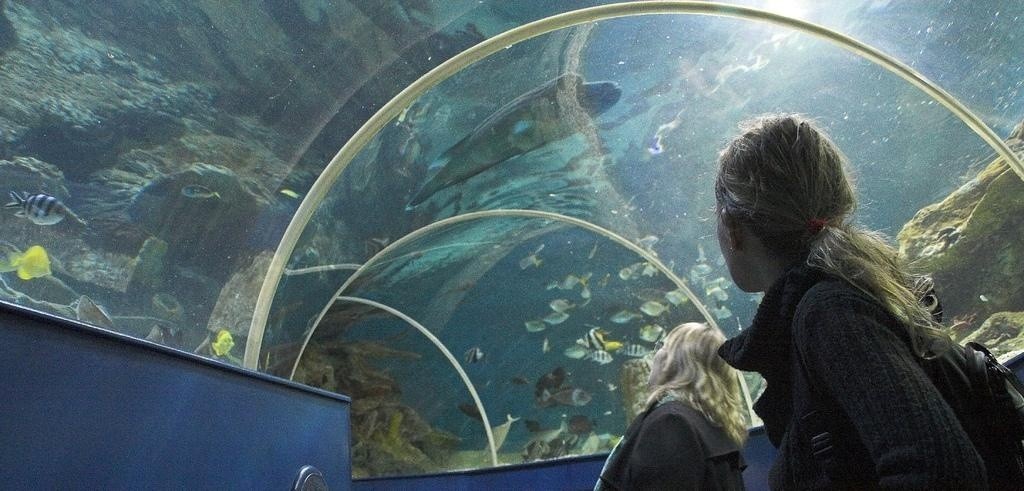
[{"left": 789, "top": 268, "right": 1024, "bottom": 491}]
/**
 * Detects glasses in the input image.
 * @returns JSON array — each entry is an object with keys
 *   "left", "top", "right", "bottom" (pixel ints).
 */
[{"left": 654, "top": 340, "right": 664, "bottom": 352}]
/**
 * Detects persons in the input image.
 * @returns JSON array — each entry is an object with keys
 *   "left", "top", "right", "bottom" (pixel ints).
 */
[
  {"left": 594, "top": 322, "right": 753, "bottom": 491},
  {"left": 709, "top": 113, "right": 1024, "bottom": 491}
]
